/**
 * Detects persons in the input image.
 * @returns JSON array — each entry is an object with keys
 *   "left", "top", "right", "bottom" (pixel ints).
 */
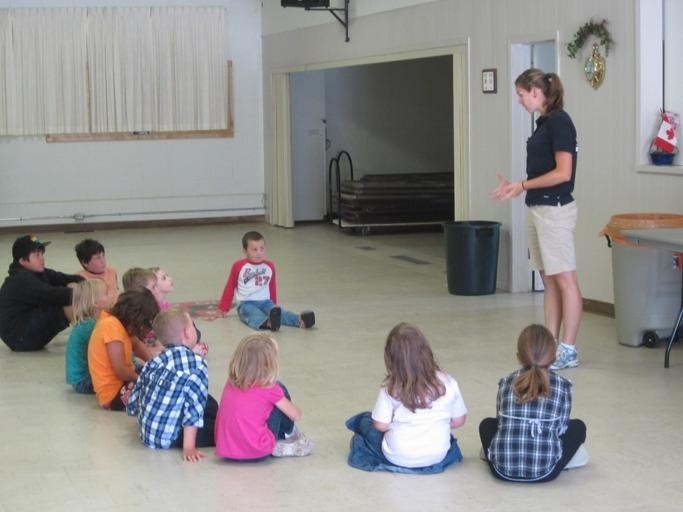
[
  {"left": 477, "top": 323, "right": 588, "bottom": 484},
  {"left": 124, "top": 305, "right": 218, "bottom": 464},
  {"left": 486, "top": 66, "right": 585, "bottom": 373},
  {"left": 343, "top": 323, "right": 469, "bottom": 474},
  {"left": 211, "top": 331, "right": 313, "bottom": 462},
  {"left": 0, "top": 233, "right": 87, "bottom": 351},
  {"left": 65, "top": 238, "right": 208, "bottom": 417},
  {"left": 209, "top": 228, "right": 316, "bottom": 331}
]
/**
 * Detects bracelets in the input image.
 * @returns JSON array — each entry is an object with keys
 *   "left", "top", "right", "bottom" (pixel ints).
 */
[{"left": 519, "top": 181, "right": 526, "bottom": 193}]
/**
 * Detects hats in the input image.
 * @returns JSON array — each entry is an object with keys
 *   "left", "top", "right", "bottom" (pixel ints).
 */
[{"left": 10, "top": 234, "right": 52, "bottom": 260}]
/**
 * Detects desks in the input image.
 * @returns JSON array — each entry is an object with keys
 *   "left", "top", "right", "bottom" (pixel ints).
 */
[{"left": 621, "top": 227, "right": 683, "bottom": 368}]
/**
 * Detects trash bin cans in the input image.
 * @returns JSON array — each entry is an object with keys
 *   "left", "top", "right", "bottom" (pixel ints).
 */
[{"left": 602, "top": 213, "right": 683, "bottom": 348}]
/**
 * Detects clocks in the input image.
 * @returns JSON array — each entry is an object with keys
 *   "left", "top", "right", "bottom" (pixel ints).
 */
[{"left": 482, "top": 68, "right": 497, "bottom": 94}]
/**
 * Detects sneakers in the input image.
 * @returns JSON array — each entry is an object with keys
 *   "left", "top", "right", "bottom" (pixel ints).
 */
[
  {"left": 547, "top": 343, "right": 580, "bottom": 370},
  {"left": 563, "top": 444, "right": 590, "bottom": 470},
  {"left": 478, "top": 442, "right": 490, "bottom": 462},
  {"left": 267, "top": 305, "right": 282, "bottom": 332},
  {"left": 269, "top": 431, "right": 316, "bottom": 459},
  {"left": 299, "top": 310, "right": 316, "bottom": 329}
]
